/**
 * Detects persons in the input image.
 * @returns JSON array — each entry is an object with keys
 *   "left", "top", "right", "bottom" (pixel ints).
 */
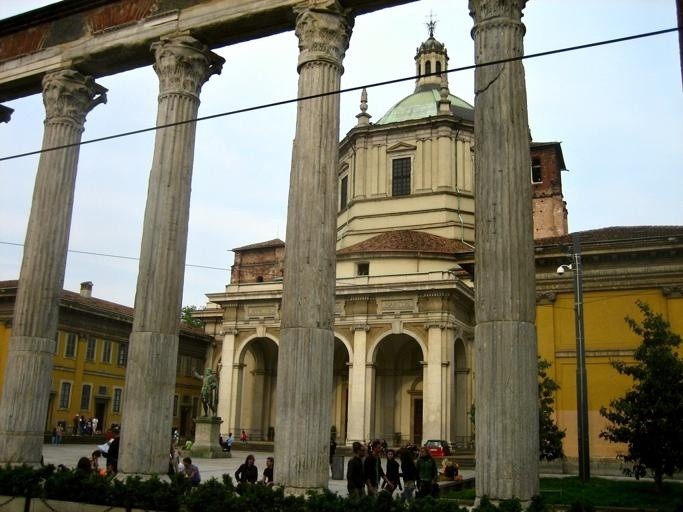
[
  {"left": 346, "top": 440, "right": 459, "bottom": 507},
  {"left": 257, "top": 457, "right": 274, "bottom": 487},
  {"left": 219, "top": 430, "right": 249, "bottom": 452},
  {"left": 330, "top": 438, "right": 337, "bottom": 472},
  {"left": 194, "top": 368, "right": 217, "bottom": 417},
  {"left": 49, "top": 413, "right": 120, "bottom": 479},
  {"left": 235, "top": 455, "right": 258, "bottom": 496},
  {"left": 168, "top": 416, "right": 201, "bottom": 496}
]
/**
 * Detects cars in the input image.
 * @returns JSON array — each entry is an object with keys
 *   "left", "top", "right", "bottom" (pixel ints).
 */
[
  {"left": 422, "top": 439, "right": 452, "bottom": 457},
  {"left": 381, "top": 444, "right": 421, "bottom": 459}
]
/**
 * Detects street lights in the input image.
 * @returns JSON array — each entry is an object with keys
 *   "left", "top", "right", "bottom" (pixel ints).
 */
[{"left": 556, "top": 253, "right": 591, "bottom": 481}]
